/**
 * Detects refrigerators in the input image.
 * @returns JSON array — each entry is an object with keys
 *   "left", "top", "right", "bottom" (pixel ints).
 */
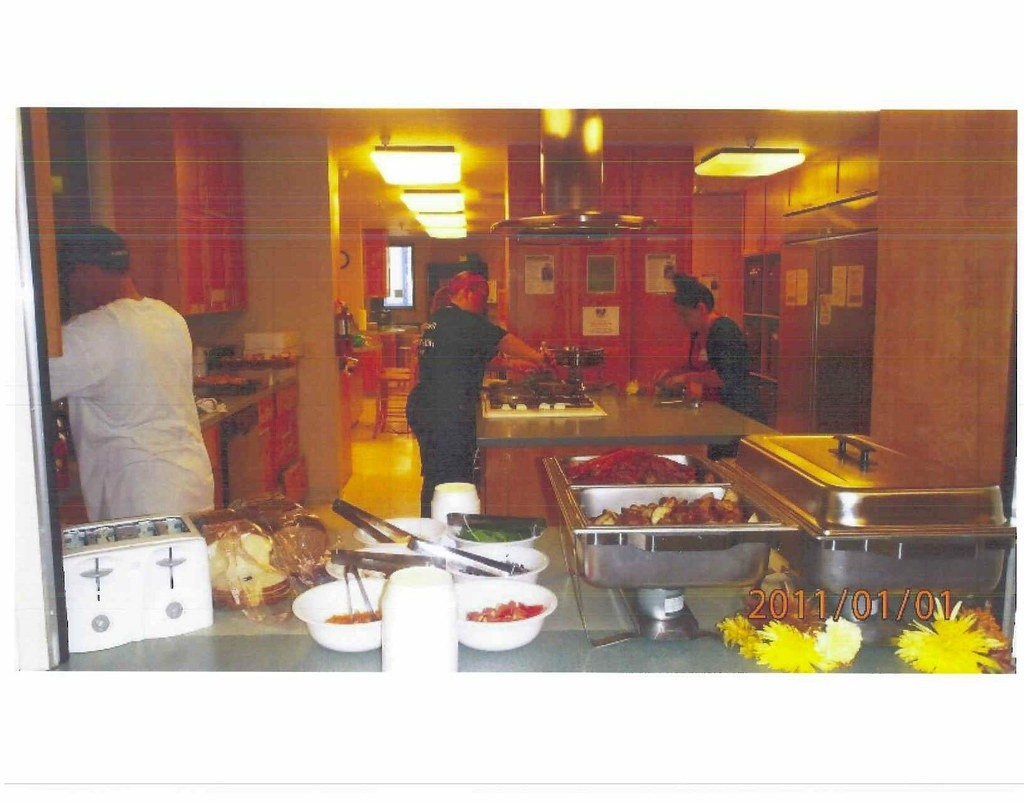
[{"left": 779, "top": 228, "right": 879, "bottom": 439}]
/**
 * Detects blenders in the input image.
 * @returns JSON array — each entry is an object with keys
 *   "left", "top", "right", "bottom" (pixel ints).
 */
[{"left": 337, "top": 305, "right": 354, "bottom": 357}]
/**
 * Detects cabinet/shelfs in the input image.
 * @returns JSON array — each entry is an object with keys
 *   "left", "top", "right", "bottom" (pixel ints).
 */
[
  {"left": 169, "top": 108, "right": 253, "bottom": 318},
  {"left": 741, "top": 168, "right": 792, "bottom": 259},
  {"left": 740, "top": 250, "right": 782, "bottom": 430},
  {"left": 788, "top": 130, "right": 880, "bottom": 215}
]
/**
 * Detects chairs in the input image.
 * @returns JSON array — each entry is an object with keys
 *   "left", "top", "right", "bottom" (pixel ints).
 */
[{"left": 370, "top": 333, "right": 422, "bottom": 442}]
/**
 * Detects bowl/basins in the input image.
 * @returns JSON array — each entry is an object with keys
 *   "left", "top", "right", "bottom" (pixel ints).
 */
[
  {"left": 454, "top": 578, "right": 560, "bottom": 652},
  {"left": 378, "top": 565, "right": 459, "bottom": 676},
  {"left": 448, "top": 522, "right": 541, "bottom": 550},
  {"left": 325, "top": 547, "right": 445, "bottom": 580},
  {"left": 291, "top": 578, "right": 388, "bottom": 652},
  {"left": 353, "top": 513, "right": 448, "bottom": 545},
  {"left": 442, "top": 545, "right": 551, "bottom": 585}
]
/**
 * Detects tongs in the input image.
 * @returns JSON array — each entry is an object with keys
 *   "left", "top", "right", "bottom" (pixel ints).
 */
[
  {"left": 344, "top": 564, "right": 377, "bottom": 625},
  {"left": 331, "top": 498, "right": 433, "bottom": 551},
  {"left": 446, "top": 512, "right": 547, "bottom": 535},
  {"left": 441, "top": 545, "right": 528, "bottom": 578},
  {"left": 329, "top": 548, "right": 445, "bottom": 577}
]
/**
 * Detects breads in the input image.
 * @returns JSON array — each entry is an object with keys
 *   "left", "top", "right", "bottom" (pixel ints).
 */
[{"left": 194, "top": 493, "right": 327, "bottom": 606}]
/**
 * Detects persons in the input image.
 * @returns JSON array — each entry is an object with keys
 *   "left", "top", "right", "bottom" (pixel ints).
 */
[
  {"left": 407, "top": 272, "right": 554, "bottom": 518},
  {"left": 50, "top": 223, "right": 216, "bottom": 523},
  {"left": 657, "top": 270, "right": 770, "bottom": 458}
]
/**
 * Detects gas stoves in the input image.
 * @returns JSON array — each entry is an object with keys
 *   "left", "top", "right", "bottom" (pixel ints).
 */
[{"left": 481, "top": 375, "right": 610, "bottom": 420}]
